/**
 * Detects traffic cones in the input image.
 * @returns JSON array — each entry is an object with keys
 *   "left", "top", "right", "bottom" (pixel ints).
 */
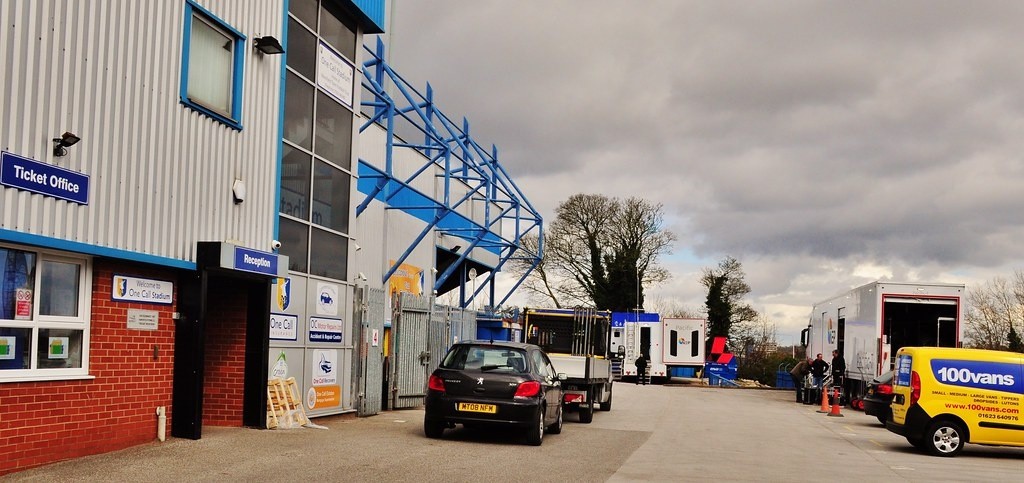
[
  {"left": 826, "top": 388, "right": 845, "bottom": 418},
  {"left": 815, "top": 386, "right": 831, "bottom": 414}
]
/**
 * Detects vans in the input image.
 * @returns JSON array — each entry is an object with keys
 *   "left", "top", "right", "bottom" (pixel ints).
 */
[{"left": 891, "top": 346, "right": 1024, "bottom": 457}]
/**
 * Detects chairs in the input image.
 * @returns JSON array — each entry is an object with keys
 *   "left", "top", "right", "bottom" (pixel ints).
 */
[
  {"left": 506, "top": 357, "right": 524, "bottom": 371},
  {"left": 471, "top": 358, "right": 484, "bottom": 368}
]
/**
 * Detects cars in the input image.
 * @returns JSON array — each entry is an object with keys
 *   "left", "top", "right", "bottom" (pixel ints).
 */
[
  {"left": 862, "top": 369, "right": 895, "bottom": 427},
  {"left": 422, "top": 339, "right": 568, "bottom": 446}
]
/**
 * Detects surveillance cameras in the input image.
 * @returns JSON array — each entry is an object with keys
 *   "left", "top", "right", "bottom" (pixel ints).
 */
[
  {"left": 272, "top": 239, "right": 282, "bottom": 251},
  {"left": 359, "top": 272, "right": 366, "bottom": 281}
]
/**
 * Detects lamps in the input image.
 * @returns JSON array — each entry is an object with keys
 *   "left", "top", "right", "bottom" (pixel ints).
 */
[
  {"left": 253, "top": 36, "right": 286, "bottom": 55},
  {"left": 450, "top": 244, "right": 461, "bottom": 253},
  {"left": 53, "top": 131, "right": 81, "bottom": 157}
]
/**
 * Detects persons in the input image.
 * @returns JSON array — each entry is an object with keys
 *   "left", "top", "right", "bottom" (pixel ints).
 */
[
  {"left": 831, "top": 350, "right": 846, "bottom": 405},
  {"left": 810, "top": 353, "right": 830, "bottom": 405},
  {"left": 635, "top": 354, "right": 647, "bottom": 386},
  {"left": 791, "top": 358, "right": 814, "bottom": 403}
]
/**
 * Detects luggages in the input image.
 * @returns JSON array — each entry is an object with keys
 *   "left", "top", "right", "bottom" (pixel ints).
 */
[{"left": 803, "top": 384, "right": 816, "bottom": 405}]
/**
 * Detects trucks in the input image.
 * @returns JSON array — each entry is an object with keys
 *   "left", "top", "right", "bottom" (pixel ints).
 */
[
  {"left": 523, "top": 306, "right": 626, "bottom": 424},
  {"left": 800, "top": 281, "right": 965, "bottom": 407}
]
